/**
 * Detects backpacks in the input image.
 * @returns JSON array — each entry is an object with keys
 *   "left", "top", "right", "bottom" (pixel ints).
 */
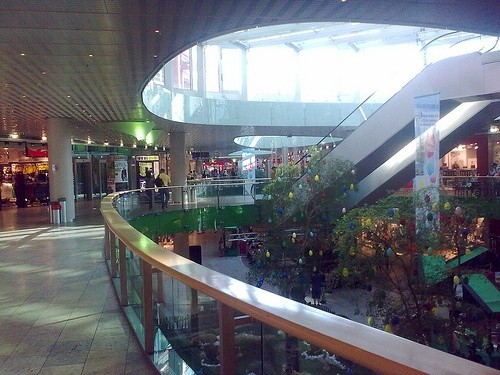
[{"left": 155, "top": 176, "right": 165, "bottom": 187}]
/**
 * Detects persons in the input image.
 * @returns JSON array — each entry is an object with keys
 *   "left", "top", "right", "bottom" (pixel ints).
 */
[
  {"left": 440, "top": 163, "right": 458, "bottom": 185},
  {"left": 311, "top": 269, "right": 326, "bottom": 306},
  {"left": 122, "top": 167, "right": 126, "bottom": 181},
  {"left": 13, "top": 170, "right": 33, "bottom": 208},
  {"left": 469, "top": 173, "right": 477, "bottom": 182},
  {"left": 187, "top": 168, "right": 238, "bottom": 191},
  {"left": 144, "top": 167, "right": 171, "bottom": 210},
  {"left": 489, "top": 163, "right": 500, "bottom": 176},
  {"left": 271, "top": 166, "right": 277, "bottom": 182}
]
